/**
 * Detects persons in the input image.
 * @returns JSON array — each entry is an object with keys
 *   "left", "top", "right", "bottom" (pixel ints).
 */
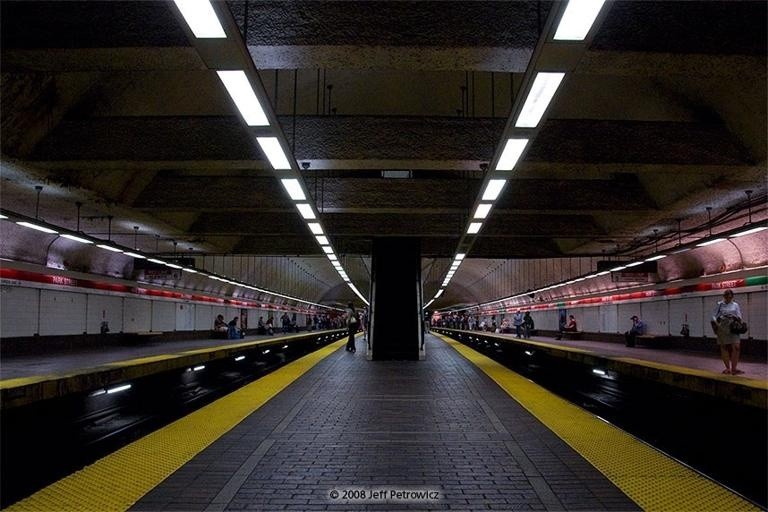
[
  {"left": 430, "top": 313, "right": 509, "bottom": 334},
  {"left": 523, "top": 311, "right": 534, "bottom": 339},
  {"left": 712, "top": 288, "right": 746, "bottom": 376},
  {"left": 210, "top": 301, "right": 369, "bottom": 354},
  {"left": 558, "top": 308, "right": 566, "bottom": 331},
  {"left": 513, "top": 310, "right": 522, "bottom": 339},
  {"left": 556, "top": 314, "right": 577, "bottom": 340},
  {"left": 622, "top": 314, "right": 644, "bottom": 348}
]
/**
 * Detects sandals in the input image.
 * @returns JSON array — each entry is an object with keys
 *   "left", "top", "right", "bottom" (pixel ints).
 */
[
  {"left": 732, "top": 368, "right": 745, "bottom": 374},
  {"left": 722, "top": 368, "right": 731, "bottom": 373}
]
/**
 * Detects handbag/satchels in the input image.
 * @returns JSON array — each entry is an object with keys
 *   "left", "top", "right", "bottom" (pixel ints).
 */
[
  {"left": 711, "top": 318, "right": 721, "bottom": 335},
  {"left": 728, "top": 319, "right": 749, "bottom": 333},
  {"left": 346, "top": 317, "right": 357, "bottom": 327}
]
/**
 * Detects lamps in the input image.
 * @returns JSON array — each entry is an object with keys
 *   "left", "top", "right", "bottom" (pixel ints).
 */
[
  {"left": 0, "top": 186, "right": 202, "bottom": 275},
  {"left": 609, "top": 192, "right": 768, "bottom": 273}
]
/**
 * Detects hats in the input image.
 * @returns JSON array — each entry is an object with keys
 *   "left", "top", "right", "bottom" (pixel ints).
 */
[{"left": 629, "top": 316, "right": 639, "bottom": 320}]
